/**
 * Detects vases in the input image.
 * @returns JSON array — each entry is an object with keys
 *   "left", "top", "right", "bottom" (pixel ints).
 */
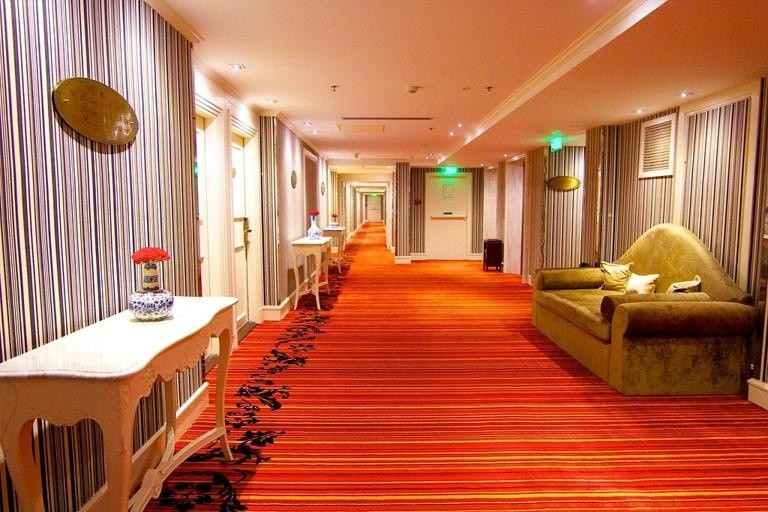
[
  {"left": 307, "top": 216, "right": 322, "bottom": 240},
  {"left": 127, "top": 264, "right": 172, "bottom": 321},
  {"left": 331, "top": 218, "right": 339, "bottom": 228}
]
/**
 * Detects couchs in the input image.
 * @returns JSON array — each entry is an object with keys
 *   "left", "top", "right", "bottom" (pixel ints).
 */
[{"left": 532, "top": 224, "right": 756, "bottom": 396}]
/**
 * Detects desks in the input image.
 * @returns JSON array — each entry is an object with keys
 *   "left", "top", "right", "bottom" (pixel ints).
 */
[
  {"left": 323, "top": 226, "right": 346, "bottom": 273},
  {"left": 0, "top": 295, "right": 238, "bottom": 512},
  {"left": 289, "top": 237, "right": 331, "bottom": 311}
]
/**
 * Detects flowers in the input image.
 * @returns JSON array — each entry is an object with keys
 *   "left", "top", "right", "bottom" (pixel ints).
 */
[
  {"left": 331, "top": 214, "right": 338, "bottom": 218},
  {"left": 307, "top": 209, "right": 320, "bottom": 215},
  {"left": 132, "top": 248, "right": 170, "bottom": 262}
]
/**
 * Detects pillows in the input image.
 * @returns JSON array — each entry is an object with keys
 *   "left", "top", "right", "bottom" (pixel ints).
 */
[
  {"left": 601, "top": 293, "right": 711, "bottom": 321},
  {"left": 668, "top": 275, "right": 702, "bottom": 292},
  {"left": 600, "top": 261, "right": 633, "bottom": 292},
  {"left": 626, "top": 272, "right": 660, "bottom": 294},
  {"left": 535, "top": 268, "right": 602, "bottom": 289}
]
